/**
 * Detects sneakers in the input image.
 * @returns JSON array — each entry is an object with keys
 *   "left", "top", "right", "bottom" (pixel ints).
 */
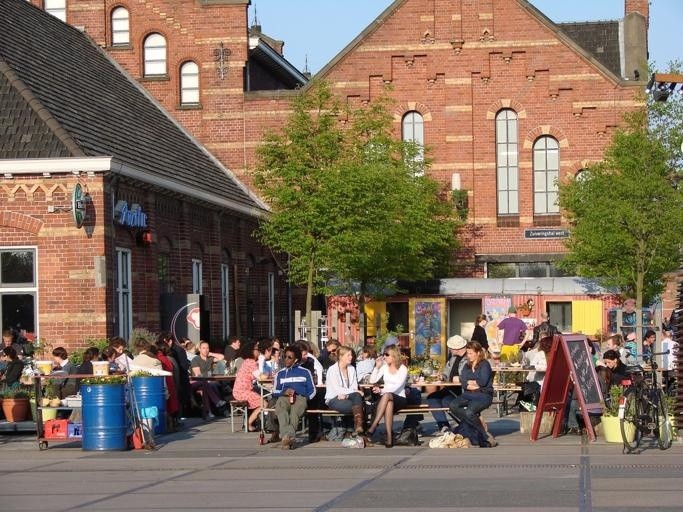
[
  {"left": 215, "top": 400, "right": 226, "bottom": 408},
  {"left": 478, "top": 416, "right": 488, "bottom": 432},
  {"left": 206, "top": 411, "right": 215, "bottom": 418},
  {"left": 519, "top": 399, "right": 536, "bottom": 411},
  {"left": 280, "top": 435, "right": 296, "bottom": 450}
]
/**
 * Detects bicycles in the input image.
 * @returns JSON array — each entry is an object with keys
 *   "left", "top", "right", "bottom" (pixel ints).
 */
[{"left": 619, "top": 350, "right": 674, "bottom": 449}]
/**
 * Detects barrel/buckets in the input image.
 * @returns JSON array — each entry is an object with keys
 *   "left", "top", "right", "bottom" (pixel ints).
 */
[
  {"left": 80, "top": 383, "right": 128, "bottom": 451},
  {"left": 132, "top": 376, "right": 164, "bottom": 436}
]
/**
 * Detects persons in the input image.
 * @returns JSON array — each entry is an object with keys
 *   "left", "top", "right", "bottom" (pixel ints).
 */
[
  {"left": 45, "top": 335, "right": 496, "bottom": 450},
  {"left": 516, "top": 310, "right": 683, "bottom": 436},
  {"left": 0, "top": 328, "right": 27, "bottom": 375},
  {"left": 496, "top": 306, "right": 529, "bottom": 363},
  {"left": 0, "top": 346, "right": 25, "bottom": 390},
  {"left": 469, "top": 311, "right": 492, "bottom": 354}
]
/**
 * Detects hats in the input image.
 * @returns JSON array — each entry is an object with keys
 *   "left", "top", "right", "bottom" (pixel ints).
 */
[
  {"left": 627, "top": 332, "right": 637, "bottom": 341},
  {"left": 508, "top": 306, "right": 518, "bottom": 314},
  {"left": 447, "top": 334, "right": 467, "bottom": 349}
]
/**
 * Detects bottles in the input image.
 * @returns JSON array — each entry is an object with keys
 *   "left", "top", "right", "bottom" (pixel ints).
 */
[
  {"left": 289, "top": 390, "right": 296, "bottom": 404},
  {"left": 419, "top": 373, "right": 424, "bottom": 382},
  {"left": 272, "top": 361, "right": 278, "bottom": 377}
]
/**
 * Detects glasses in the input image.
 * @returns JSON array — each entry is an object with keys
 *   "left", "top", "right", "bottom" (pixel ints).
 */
[
  {"left": 283, "top": 355, "right": 292, "bottom": 359},
  {"left": 328, "top": 347, "right": 338, "bottom": 354},
  {"left": 382, "top": 352, "right": 390, "bottom": 357}
]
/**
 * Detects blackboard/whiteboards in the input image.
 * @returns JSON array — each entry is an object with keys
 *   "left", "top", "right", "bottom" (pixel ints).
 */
[{"left": 562, "top": 335, "right": 605, "bottom": 410}]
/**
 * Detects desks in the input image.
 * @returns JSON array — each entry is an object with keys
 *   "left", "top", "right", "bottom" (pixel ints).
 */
[{"left": 643, "top": 366, "right": 667, "bottom": 372}]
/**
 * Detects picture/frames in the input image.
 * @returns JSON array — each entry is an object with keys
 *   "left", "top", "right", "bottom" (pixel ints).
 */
[{"left": 407, "top": 294, "right": 448, "bottom": 368}]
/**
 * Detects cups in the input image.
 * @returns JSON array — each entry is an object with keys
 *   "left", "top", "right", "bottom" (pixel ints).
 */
[{"left": 207, "top": 370, "right": 212, "bottom": 377}]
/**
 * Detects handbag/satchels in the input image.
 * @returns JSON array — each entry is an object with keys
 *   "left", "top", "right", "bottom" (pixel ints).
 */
[
  {"left": 381, "top": 427, "right": 419, "bottom": 446},
  {"left": 429, "top": 432, "right": 471, "bottom": 448}
]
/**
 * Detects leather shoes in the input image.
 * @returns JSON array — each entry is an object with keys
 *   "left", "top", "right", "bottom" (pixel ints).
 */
[
  {"left": 365, "top": 428, "right": 376, "bottom": 436},
  {"left": 386, "top": 441, "right": 393, "bottom": 448}
]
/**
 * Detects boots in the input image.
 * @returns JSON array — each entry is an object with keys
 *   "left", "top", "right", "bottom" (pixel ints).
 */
[
  {"left": 352, "top": 404, "right": 364, "bottom": 432},
  {"left": 267, "top": 432, "right": 280, "bottom": 442}
]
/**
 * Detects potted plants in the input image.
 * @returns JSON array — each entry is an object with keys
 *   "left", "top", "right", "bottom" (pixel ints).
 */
[
  {"left": 0, "top": 324, "right": 168, "bottom": 452},
  {"left": 517, "top": 302, "right": 532, "bottom": 316},
  {"left": 600, "top": 386, "right": 636, "bottom": 444},
  {"left": 649, "top": 395, "right": 678, "bottom": 442}
]
